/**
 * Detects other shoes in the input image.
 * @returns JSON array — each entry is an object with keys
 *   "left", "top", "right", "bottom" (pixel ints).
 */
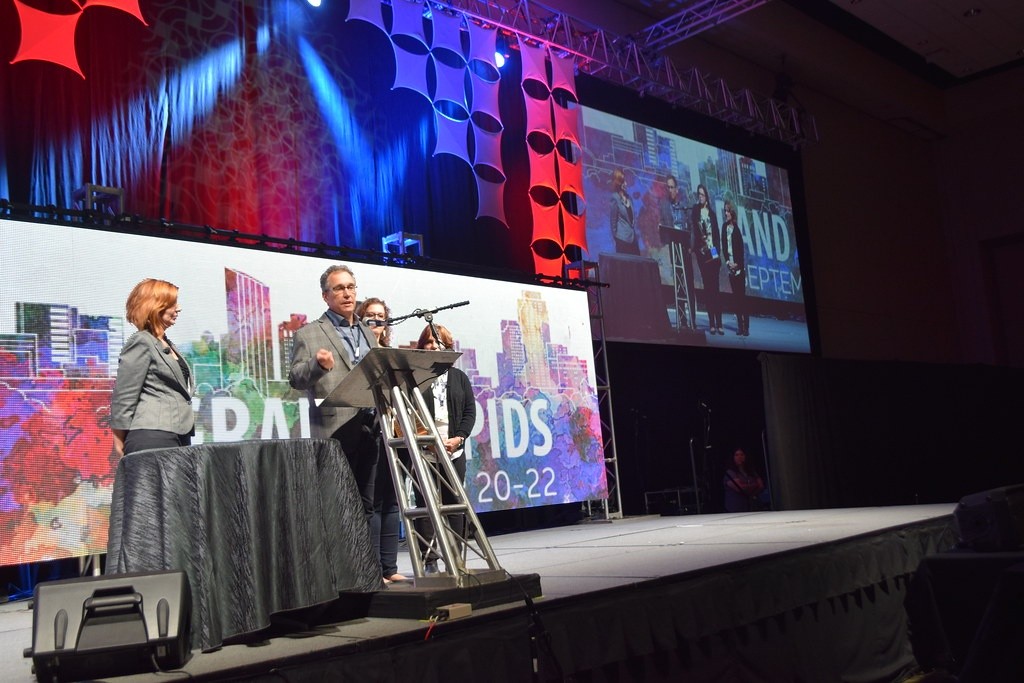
[
  {"left": 743, "top": 330, "right": 749, "bottom": 336},
  {"left": 718, "top": 329, "right": 724, "bottom": 335},
  {"left": 736, "top": 329, "right": 743, "bottom": 335},
  {"left": 425, "top": 561, "right": 440, "bottom": 573},
  {"left": 710, "top": 328, "right": 716, "bottom": 334},
  {"left": 383, "top": 576, "right": 414, "bottom": 585}
]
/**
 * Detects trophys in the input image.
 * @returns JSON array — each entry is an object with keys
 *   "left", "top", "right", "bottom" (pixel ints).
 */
[{"left": 694, "top": 220, "right": 719, "bottom": 264}]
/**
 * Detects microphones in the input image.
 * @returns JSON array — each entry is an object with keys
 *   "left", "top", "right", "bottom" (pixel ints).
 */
[
  {"left": 673, "top": 204, "right": 679, "bottom": 210},
  {"left": 362, "top": 317, "right": 387, "bottom": 327},
  {"left": 702, "top": 403, "right": 712, "bottom": 413},
  {"left": 163, "top": 347, "right": 171, "bottom": 354}
]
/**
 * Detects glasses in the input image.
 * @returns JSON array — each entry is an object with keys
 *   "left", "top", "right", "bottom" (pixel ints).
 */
[
  {"left": 668, "top": 186, "right": 676, "bottom": 188},
  {"left": 329, "top": 285, "right": 357, "bottom": 293},
  {"left": 366, "top": 312, "right": 386, "bottom": 318}
]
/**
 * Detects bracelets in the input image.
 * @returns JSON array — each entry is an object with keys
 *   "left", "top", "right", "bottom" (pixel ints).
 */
[{"left": 460, "top": 436, "right": 465, "bottom": 446}]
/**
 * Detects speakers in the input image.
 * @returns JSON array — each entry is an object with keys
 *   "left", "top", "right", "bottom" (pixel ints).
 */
[
  {"left": 955, "top": 485, "right": 1024, "bottom": 549},
  {"left": 22, "top": 570, "right": 193, "bottom": 683}
]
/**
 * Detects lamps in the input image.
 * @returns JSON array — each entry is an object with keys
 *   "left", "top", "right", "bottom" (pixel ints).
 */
[{"left": 495, "top": 39, "right": 508, "bottom": 67}]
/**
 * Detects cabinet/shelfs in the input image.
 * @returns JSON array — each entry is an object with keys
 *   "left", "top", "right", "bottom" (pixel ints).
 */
[
  {"left": 382, "top": 231, "right": 424, "bottom": 265},
  {"left": 70, "top": 183, "right": 125, "bottom": 222}
]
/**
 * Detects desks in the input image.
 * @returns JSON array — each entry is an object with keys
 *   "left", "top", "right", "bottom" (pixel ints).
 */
[{"left": 105, "top": 439, "right": 386, "bottom": 654}]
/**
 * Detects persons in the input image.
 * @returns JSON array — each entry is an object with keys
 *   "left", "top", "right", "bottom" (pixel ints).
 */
[
  {"left": 410, "top": 324, "right": 475, "bottom": 573},
  {"left": 659, "top": 174, "right": 750, "bottom": 336},
  {"left": 111, "top": 279, "right": 195, "bottom": 457},
  {"left": 289, "top": 266, "right": 380, "bottom": 542},
  {"left": 355, "top": 298, "right": 412, "bottom": 584},
  {"left": 718, "top": 446, "right": 764, "bottom": 513},
  {"left": 610, "top": 169, "right": 640, "bottom": 255}
]
